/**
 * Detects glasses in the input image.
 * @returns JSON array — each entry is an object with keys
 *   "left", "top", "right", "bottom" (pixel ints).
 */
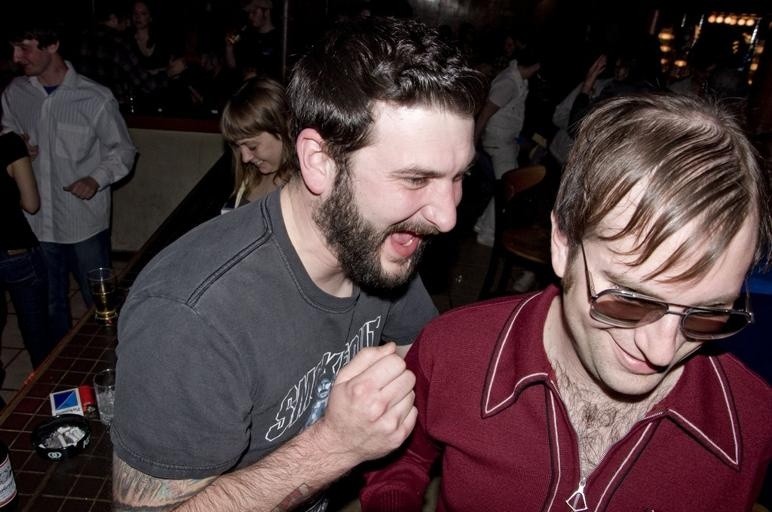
[{"left": 578, "top": 239, "right": 755, "bottom": 342}]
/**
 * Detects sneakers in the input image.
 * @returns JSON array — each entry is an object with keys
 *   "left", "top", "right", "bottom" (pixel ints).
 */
[
  {"left": 513, "top": 270, "right": 536, "bottom": 292},
  {"left": 474, "top": 224, "right": 495, "bottom": 248}
]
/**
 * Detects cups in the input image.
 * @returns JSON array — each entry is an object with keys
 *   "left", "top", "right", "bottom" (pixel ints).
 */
[
  {"left": 83, "top": 267, "right": 119, "bottom": 323},
  {"left": 93, "top": 366, "right": 117, "bottom": 426}
]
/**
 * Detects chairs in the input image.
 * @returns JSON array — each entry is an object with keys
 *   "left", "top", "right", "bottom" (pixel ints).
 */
[
  {"left": 419, "top": 172, "right": 492, "bottom": 310},
  {"left": 479, "top": 165, "right": 550, "bottom": 299}
]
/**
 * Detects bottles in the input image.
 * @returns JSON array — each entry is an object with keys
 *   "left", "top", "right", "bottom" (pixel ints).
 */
[
  {"left": 228, "top": 24, "right": 248, "bottom": 46},
  {"left": 0, "top": 438, "right": 20, "bottom": 512}
]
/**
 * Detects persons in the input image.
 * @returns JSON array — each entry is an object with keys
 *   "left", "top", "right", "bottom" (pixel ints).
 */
[
  {"left": 359, "top": 88, "right": 769, "bottom": 511},
  {"left": 110, "top": 8, "right": 480, "bottom": 511},
  {"left": 2, "top": 2, "right": 138, "bottom": 342},
  {"left": 87, "top": 2, "right": 306, "bottom": 123},
  {"left": 430, "top": 12, "right": 770, "bottom": 300},
  {"left": 1, "top": 108, "right": 53, "bottom": 376},
  {"left": 217, "top": 76, "right": 300, "bottom": 220}
]
